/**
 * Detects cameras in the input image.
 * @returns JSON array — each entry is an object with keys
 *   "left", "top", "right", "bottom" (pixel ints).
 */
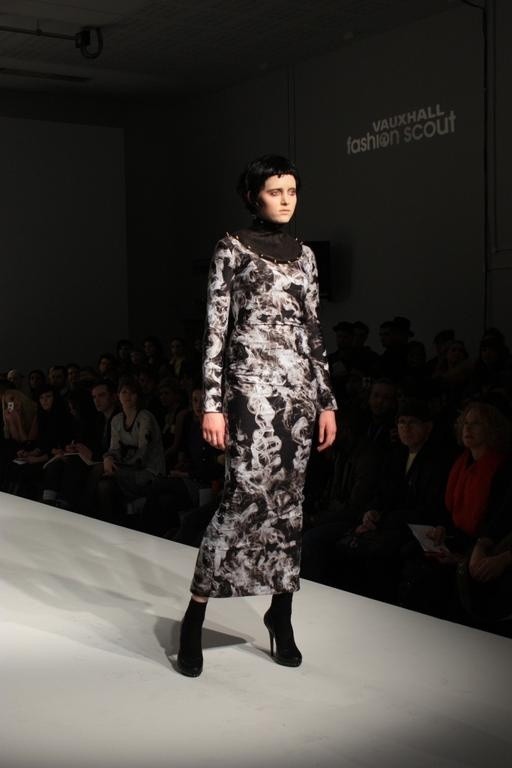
[{"left": 7, "top": 402, "right": 15, "bottom": 413}]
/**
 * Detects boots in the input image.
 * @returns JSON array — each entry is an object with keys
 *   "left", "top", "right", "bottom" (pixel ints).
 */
[
  {"left": 263, "top": 592, "right": 302, "bottom": 668},
  {"left": 177, "top": 598, "right": 207, "bottom": 677}
]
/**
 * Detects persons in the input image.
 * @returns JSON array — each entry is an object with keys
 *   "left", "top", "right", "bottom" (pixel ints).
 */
[
  {"left": 174, "top": 154, "right": 342, "bottom": 679},
  {"left": 0, "top": 313, "right": 512, "bottom": 642}
]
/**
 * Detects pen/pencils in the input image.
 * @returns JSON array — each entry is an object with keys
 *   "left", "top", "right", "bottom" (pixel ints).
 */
[
  {"left": 71, "top": 439, "right": 75, "bottom": 444},
  {"left": 444, "top": 535, "right": 454, "bottom": 538}
]
